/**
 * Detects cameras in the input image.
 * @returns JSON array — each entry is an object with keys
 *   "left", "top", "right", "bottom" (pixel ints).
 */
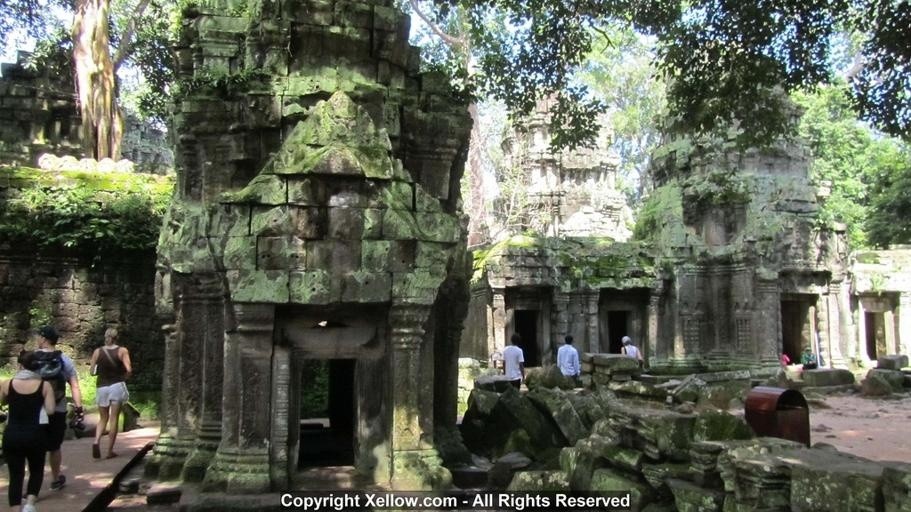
[{"left": 68, "top": 410, "right": 85, "bottom": 431}]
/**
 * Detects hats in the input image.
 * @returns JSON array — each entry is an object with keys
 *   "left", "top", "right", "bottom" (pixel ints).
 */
[{"left": 38, "top": 326, "right": 62, "bottom": 339}]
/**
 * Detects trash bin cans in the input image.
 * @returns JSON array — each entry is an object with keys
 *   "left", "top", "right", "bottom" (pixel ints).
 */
[{"left": 745, "top": 386, "right": 810, "bottom": 448}]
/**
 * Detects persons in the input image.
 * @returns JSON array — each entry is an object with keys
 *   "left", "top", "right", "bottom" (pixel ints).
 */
[
  {"left": 0, "top": 350, "right": 56, "bottom": 512},
  {"left": 501, "top": 333, "right": 524, "bottom": 390},
  {"left": 801, "top": 346, "right": 818, "bottom": 370},
  {"left": 89, "top": 327, "right": 133, "bottom": 459},
  {"left": 557, "top": 335, "right": 581, "bottom": 377},
  {"left": 21, "top": 325, "right": 84, "bottom": 502},
  {"left": 621, "top": 336, "right": 643, "bottom": 365},
  {"left": 782, "top": 354, "right": 790, "bottom": 365}
]
